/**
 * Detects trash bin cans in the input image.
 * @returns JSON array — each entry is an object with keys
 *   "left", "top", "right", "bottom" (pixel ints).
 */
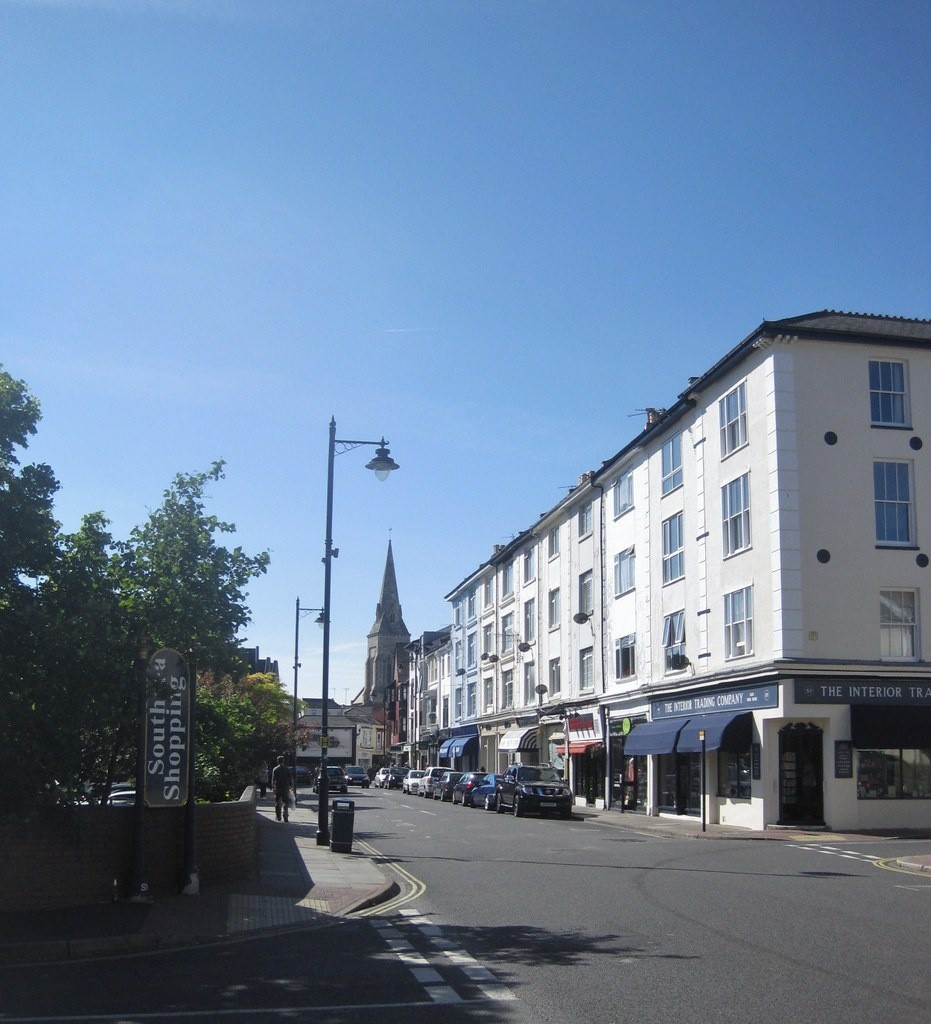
[{"left": 329, "top": 798, "right": 356, "bottom": 854}]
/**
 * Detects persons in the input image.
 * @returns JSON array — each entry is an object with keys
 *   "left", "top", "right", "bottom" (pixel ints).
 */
[
  {"left": 367, "top": 761, "right": 409, "bottom": 790},
  {"left": 258, "top": 768, "right": 269, "bottom": 798},
  {"left": 272, "top": 756, "right": 296, "bottom": 822}
]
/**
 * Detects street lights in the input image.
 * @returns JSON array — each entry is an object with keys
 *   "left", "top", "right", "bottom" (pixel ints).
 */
[
  {"left": 291, "top": 596, "right": 332, "bottom": 805},
  {"left": 315, "top": 413, "right": 401, "bottom": 846}
]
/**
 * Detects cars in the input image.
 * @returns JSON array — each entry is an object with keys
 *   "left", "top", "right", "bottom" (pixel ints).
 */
[
  {"left": 291, "top": 766, "right": 311, "bottom": 785},
  {"left": 452, "top": 772, "right": 489, "bottom": 806},
  {"left": 402, "top": 770, "right": 425, "bottom": 795},
  {"left": 432, "top": 772, "right": 465, "bottom": 801},
  {"left": 417, "top": 766, "right": 457, "bottom": 798},
  {"left": 470, "top": 774, "right": 503, "bottom": 810},
  {"left": 375, "top": 768, "right": 390, "bottom": 788},
  {"left": 313, "top": 765, "right": 347, "bottom": 794},
  {"left": 344, "top": 766, "right": 369, "bottom": 788}
]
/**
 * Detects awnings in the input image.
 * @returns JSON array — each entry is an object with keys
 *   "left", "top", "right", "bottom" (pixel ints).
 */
[
  {"left": 498, "top": 726, "right": 543, "bottom": 752},
  {"left": 556, "top": 742, "right": 602, "bottom": 753},
  {"left": 623, "top": 718, "right": 691, "bottom": 755},
  {"left": 677, "top": 711, "right": 753, "bottom": 753},
  {"left": 439, "top": 734, "right": 478, "bottom": 759}
]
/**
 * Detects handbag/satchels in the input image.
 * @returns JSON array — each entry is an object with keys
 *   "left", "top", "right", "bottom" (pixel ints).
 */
[{"left": 288, "top": 788, "right": 295, "bottom": 808}]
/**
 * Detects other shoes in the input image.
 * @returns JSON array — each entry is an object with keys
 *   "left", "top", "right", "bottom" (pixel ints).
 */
[
  {"left": 275, "top": 817, "right": 281, "bottom": 821},
  {"left": 284, "top": 818, "right": 288, "bottom": 822}
]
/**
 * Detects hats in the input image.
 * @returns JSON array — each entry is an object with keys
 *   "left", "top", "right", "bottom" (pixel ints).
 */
[{"left": 277, "top": 756, "right": 284, "bottom": 764}]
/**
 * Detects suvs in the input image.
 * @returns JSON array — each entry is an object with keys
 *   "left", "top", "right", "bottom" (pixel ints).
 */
[
  {"left": 383, "top": 767, "right": 410, "bottom": 789},
  {"left": 496, "top": 762, "right": 573, "bottom": 817}
]
